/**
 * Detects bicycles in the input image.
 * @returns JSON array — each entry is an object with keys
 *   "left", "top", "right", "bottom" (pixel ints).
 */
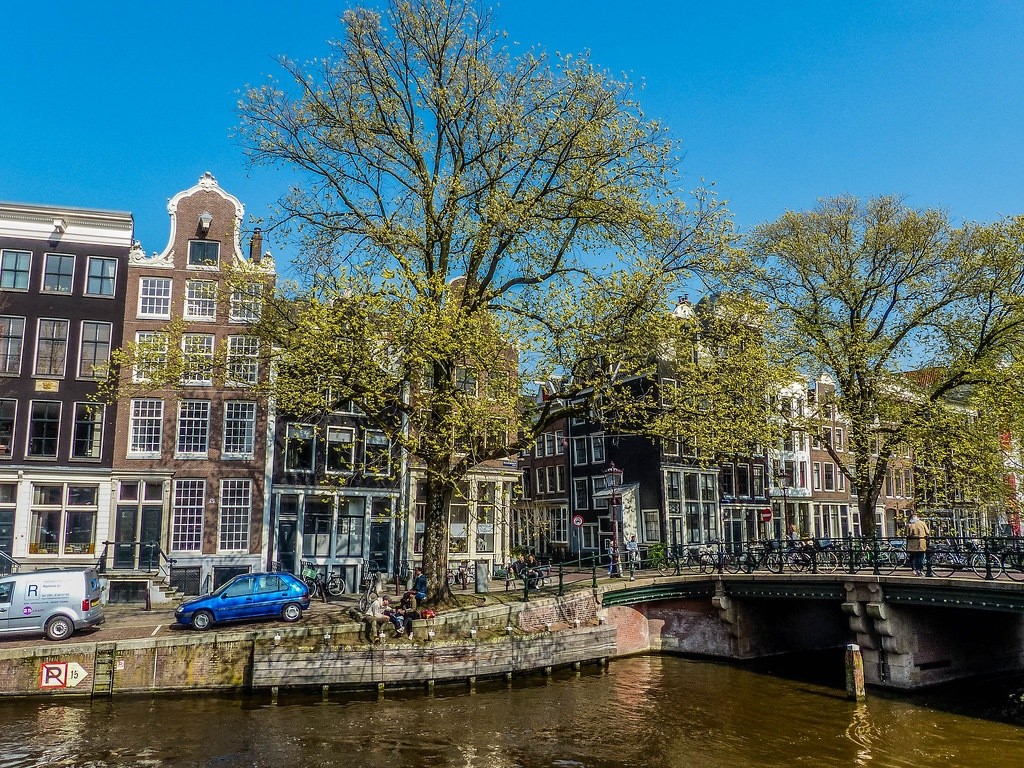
[
  {"left": 304, "top": 572, "right": 346, "bottom": 598},
  {"left": 359, "top": 574, "right": 380, "bottom": 614},
  {"left": 655, "top": 528, "right": 1023, "bottom": 584},
  {"left": 446, "top": 567, "right": 471, "bottom": 586}
]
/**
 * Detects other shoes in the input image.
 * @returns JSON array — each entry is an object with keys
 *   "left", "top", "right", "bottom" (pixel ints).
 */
[
  {"left": 396, "top": 627, "right": 404, "bottom": 634},
  {"left": 912, "top": 570, "right": 924, "bottom": 577},
  {"left": 374, "top": 636, "right": 379, "bottom": 643},
  {"left": 377, "top": 630, "right": 383, "bottom": 635},
  {"left": 409, "top": 633, "right": 414, "bottom": 640}
]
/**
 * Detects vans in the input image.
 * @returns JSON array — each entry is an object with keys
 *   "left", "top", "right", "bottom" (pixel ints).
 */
[{"left": 0, "top": 567, "right": 105, "bottom": 642}]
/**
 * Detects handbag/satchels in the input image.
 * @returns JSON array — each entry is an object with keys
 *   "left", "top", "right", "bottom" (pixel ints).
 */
[{"left": 421, "top": 610, "right": 435, "bottom": 619}]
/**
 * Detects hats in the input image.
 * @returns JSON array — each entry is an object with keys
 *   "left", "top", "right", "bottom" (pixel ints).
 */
[{"left": 383, "top": 595, "right": 392, "bottom": 602}]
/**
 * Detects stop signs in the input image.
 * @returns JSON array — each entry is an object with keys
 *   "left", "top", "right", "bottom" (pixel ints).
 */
[{"left": 762, "top": 509, "right": 771, "bottom": 522}]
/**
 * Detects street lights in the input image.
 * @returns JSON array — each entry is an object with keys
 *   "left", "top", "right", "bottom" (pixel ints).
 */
[{"left": 600, "top": 460, "right": 624, "bottom": 579}]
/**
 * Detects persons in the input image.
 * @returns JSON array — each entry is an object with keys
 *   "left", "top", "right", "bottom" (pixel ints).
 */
[
  {"left": 626, "top": 536, "right": 639, "bottom": 573},
  {"left": 607, "top": 541, "right": 616, "bottom": 574},
  {"left": 365, "top": 595, "right": 393, "bottom": 644},
  {"left": 904, "top": 513, "right": 929, "bottom": 577},
  {"left": 527, "top": 555, "right": 544, "bottom": 586},
  {"left": 395, "top": 593, "right": 417, "bottom": 641},
  {"left": 410, "top": 567, "right": 426, "bottom": 600},
  {"left": 509, "top": 555, "right": 540, "bottom": 590}
]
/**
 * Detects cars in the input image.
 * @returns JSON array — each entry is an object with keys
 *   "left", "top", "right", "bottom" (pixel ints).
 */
[{"left": 174, "top": 572, "right": 312, "bottom": 631}]
